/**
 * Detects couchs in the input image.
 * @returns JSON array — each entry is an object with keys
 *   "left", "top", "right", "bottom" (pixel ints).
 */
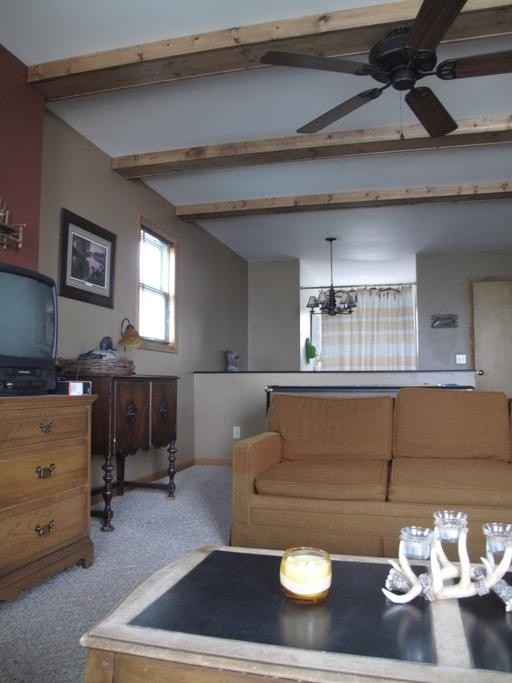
[{"left": 225, "top": 391, "right": 512, "bottom": 562}]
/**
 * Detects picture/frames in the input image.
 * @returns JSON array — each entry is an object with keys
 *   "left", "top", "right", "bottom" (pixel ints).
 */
[{"left": 54, "top": 204, "right": 118, "bottom": 310}]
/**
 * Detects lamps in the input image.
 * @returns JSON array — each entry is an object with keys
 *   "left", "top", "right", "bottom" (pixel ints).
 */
[
  {"left": 304, "top": 236, "right": 357, "bottom": 317},
  {"left": 116, "top": 316, "right": 144, "bottom": 360}
]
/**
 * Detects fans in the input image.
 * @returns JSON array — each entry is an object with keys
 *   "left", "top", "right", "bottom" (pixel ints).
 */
[{"left": 258, "top": 0, "right": 510, "bottom": 139}]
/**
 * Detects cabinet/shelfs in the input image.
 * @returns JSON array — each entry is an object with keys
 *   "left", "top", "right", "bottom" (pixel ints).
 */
[
  {"left": 53, "top": 372, "right": 180, "bottom": 533},
  {"left": 0, "top": 390, "right": 99, "bottom": 600}
]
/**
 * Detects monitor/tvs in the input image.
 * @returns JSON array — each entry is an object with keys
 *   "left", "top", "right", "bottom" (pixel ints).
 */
[{"left": 0, "top": 262, "right": 58, "bottom": 396}]
